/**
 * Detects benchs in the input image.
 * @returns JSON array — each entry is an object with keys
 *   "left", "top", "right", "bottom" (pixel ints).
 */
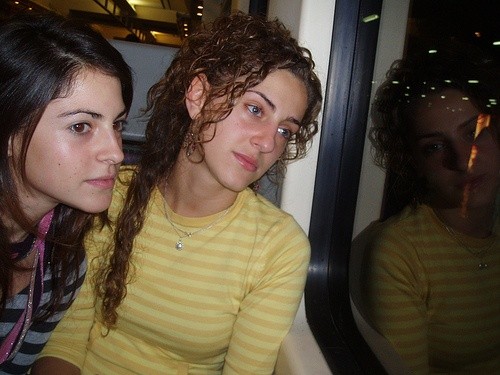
[{"left": 119, "top": 153, "right": 142, "bottom": 167}]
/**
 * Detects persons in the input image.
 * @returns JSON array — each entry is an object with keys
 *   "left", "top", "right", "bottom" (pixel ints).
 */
[
  {"left": 27, "top": 8, "right": 325, "bottom": 374},
  {"left": 357, "top": 54, "right": 499, "bottom": 375},
  {"left": 0, "top": 0, "right": 136, "bottom": 375}
]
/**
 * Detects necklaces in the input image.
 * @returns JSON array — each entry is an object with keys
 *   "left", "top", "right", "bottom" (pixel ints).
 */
[
  {"left": 162, "top": 158, "right": 241, "bottom": 250},
  {"left": 423, "top": 198, "right": 500, "bottom": 270}
]
